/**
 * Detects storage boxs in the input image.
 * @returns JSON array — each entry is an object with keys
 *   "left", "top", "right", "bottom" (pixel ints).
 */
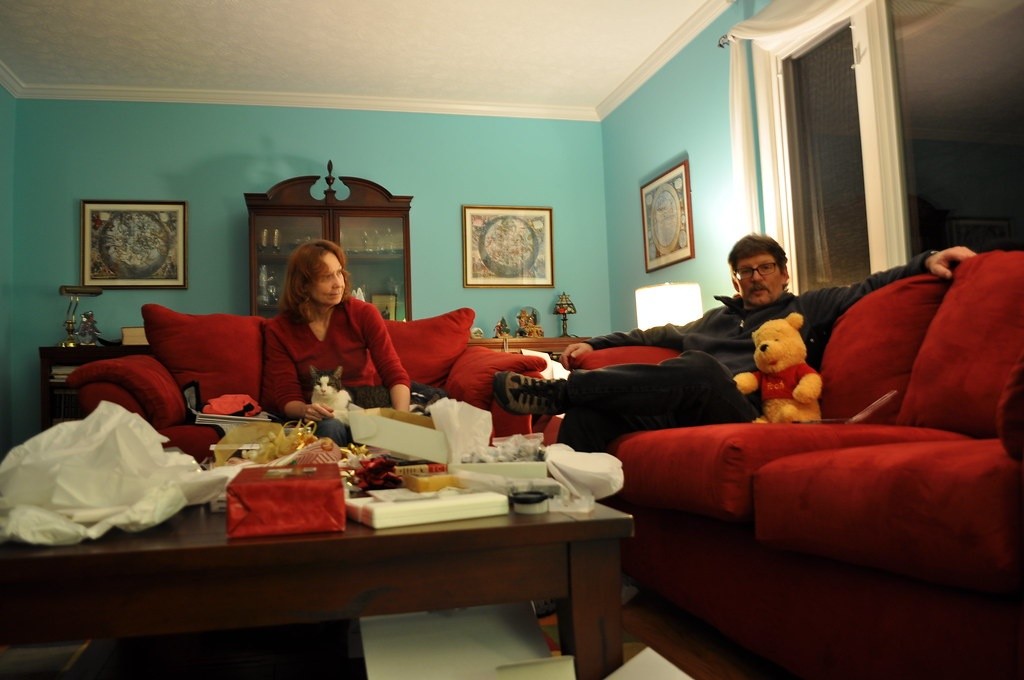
[
  {"left": 349, "top": 409, "right": 449, "bottom": 465},
  {"left": 227, "top": 463, "right": 346, "bottom": 536},
  {"left": 406, "top": 475, "right": 466, "bottom": 493},
  {"left": 449, "top": 460, "right": 546, "bottom": 482}
]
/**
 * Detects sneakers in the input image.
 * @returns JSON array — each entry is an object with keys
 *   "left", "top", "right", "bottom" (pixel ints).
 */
[{"left": 494, "top": 370, "right": 568, "bottom": 416}]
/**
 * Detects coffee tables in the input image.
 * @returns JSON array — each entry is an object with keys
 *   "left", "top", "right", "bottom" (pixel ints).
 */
[{"left": 0, "top": 504, "right": 633, "bottom": 680}]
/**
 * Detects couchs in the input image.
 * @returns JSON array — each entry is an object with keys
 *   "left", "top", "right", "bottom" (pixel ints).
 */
[
  {"left": 66, "top": 303, "right": 546, "bottom": 466},
  {"left": 574, "top": 251, "right": 1024, "bottom": 680}
]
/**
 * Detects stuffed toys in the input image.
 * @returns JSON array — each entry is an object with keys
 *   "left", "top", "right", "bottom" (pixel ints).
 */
[{"left": 734, "top": 312, "right": 822, "bottom": 424}]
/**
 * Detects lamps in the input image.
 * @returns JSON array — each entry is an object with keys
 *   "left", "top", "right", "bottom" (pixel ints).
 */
[
  {"left": 554, "top": 292, "right": 576, "bottom": 339},
  {"left": 58, "top": 285, "right": 103, "bottom": 346}
]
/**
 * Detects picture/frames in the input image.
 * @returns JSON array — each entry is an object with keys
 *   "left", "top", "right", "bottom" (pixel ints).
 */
[
  {"left": 638, "top": 161, "right": 694, "bottom": 270},
  {"left": 463, "top": 205, "right": 555, "bottom": 289},
  {"left": 79, "top": 199, "right": 187, "bottom": 290}
]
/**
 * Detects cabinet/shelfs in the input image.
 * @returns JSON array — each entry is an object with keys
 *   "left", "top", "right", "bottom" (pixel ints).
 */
[
  {"left": 467, "top": 336, "right": 591, "bottom": 358},
  {"left": 243, "top": 162, "right": 411, "bottom": 329},
  {"left": 37, "top": 347, "right": 151, "bottom": 435}
]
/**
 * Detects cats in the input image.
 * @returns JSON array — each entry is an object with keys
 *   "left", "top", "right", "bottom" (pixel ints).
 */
[{"left": 309, "top": 364, "right": 448, "bottom": 427}]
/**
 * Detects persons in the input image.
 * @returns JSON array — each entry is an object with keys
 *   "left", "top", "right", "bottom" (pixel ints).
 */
[
  {"left": 265, "top": 240, "right": 410, "bottom": 447},
  {"left": 493, "top": 234, "right": 978, "bottom": 619}
]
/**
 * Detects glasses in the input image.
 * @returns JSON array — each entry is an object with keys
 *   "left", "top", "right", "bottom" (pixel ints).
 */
[{"left": 733, "top": 262, "right": 777, "bottom": 281}]
